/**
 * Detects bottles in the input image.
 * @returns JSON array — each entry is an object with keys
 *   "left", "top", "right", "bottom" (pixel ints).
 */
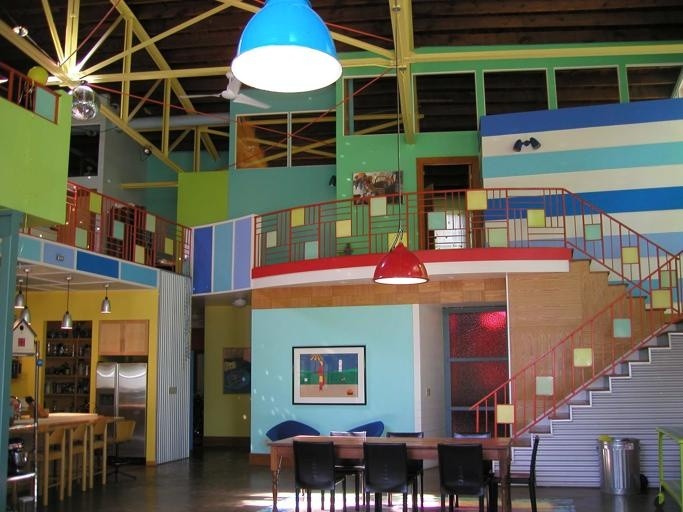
[{"left": 45, "top": 340, "right": 75, "bottom": 358}]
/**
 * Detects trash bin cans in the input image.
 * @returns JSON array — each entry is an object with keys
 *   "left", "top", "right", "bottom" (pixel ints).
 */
[{"left": 599, "top": 437, "right": 641, "bottom": 496}]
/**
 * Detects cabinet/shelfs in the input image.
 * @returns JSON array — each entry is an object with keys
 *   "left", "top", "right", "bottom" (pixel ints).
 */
[
  {"left": 44, "top": 321, "right": 91, "bottom": 413},
  {"left": 98, "top": 320, "right": 149, "bottom": 356},
  {"left": 655, "top": 426, "right": 683, "bottom": 512}
]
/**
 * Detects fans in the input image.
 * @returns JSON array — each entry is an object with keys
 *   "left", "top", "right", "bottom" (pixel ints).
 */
[{"left": 178, "top": 70, "right": 271, "bottom": 109}]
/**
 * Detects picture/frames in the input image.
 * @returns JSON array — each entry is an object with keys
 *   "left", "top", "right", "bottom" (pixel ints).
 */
[{"left": 291, "top": 345, "right": 367, "bottom": 406}]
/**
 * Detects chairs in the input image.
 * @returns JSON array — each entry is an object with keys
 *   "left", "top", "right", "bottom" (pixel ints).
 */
[
  {"left": 22, "top": 420, "right": 137, "bottom": 506},
  {"left": 292, "top": 432, "right": 540, "bottom": 512}
]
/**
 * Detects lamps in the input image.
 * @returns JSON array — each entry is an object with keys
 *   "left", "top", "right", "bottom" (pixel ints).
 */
[
  {"left": 232, "top": 298, "right": 247, "bottom": 307},
  {"left": 143, "top": 147, "right": 152, "bottom": 156},
  {"left": 328, "top": 175, "right": 336, "bottom": 188},
  {"left": 529, "top": 136, "right": 542, "bottom": 150},
  {"left": 15, "top": 268, "right": 111, "bottom": 329},
  {"left": 231, "top": 0, "right": 343, "bottom": 93},
  {"left": 513, "top": 138, "right": 523, "bottom": 152},
  {"left": 68, "top": 81, "right": 101, "bottom": 120},
  {"left": 373, "top": 0, "right": 429, "bottom": 285}
]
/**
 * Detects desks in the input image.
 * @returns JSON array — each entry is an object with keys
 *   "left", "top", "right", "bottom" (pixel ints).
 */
[{"left": 267, "top": 435, "right": 512, "bottom": 512}]
